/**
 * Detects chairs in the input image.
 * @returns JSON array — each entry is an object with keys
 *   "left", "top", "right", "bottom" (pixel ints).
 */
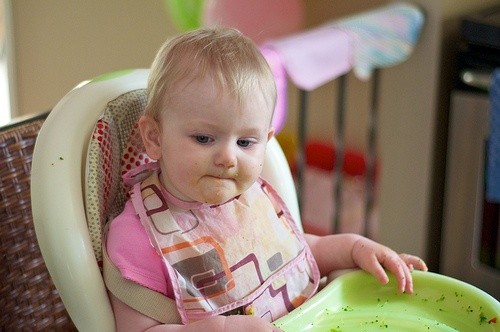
[{"left": 30, "top": 65, "right": 500, "bottom": 332}]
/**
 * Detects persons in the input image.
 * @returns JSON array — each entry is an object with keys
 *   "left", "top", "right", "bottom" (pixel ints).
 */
[{"left": 101, "top": 25, "right": 429, "bottom": 332}]
